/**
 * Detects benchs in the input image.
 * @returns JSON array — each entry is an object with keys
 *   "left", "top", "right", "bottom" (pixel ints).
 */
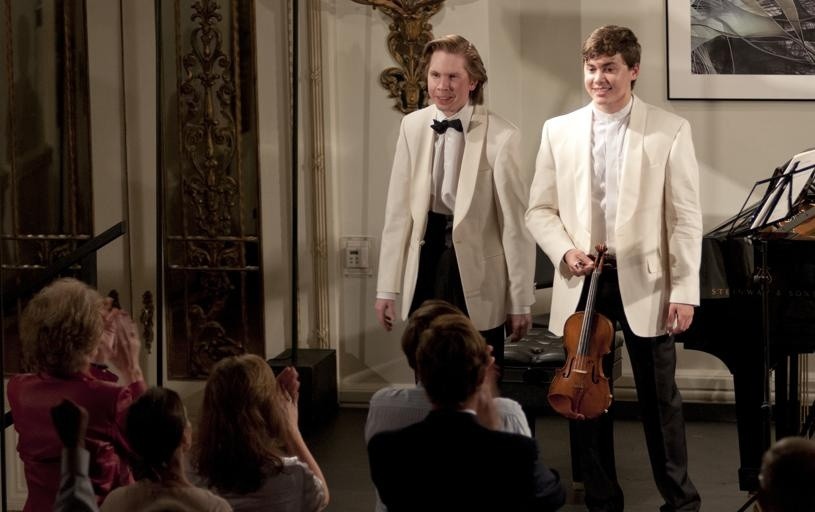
[{"left": 498, "top": 326, "right": 625, "bottom": 439}]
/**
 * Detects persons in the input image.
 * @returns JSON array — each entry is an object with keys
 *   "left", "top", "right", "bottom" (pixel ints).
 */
[
  {"left": 365, "top": 297, "right": 533, "bottom": 512},
  {"left": 0, "top": 275, "right": 147, "bottom": 512},
  {"left": 99, "top": 386, "right": 233, "bottom": 512},
  {"left": 45, "top": 395, "right": 100, "bottom": 512},
  {"left": 188, "top": 352, "right": 331, "bottom": 512},
  {"left": 366, "top": 31, "right": 538, "bottom": 395},
  {"left": 366, "top": 312, "right": 567, "bottom": 510},
  {"left": 755, "top": 434, "right": 814, "bottom": 512},
  {"left": 524, "top": 21, "right": 706, "bottom": 510}
]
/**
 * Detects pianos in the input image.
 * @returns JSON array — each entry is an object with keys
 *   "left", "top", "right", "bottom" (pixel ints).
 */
[{"left": 674, "top": 149, "right": 815, "bottom": 492}]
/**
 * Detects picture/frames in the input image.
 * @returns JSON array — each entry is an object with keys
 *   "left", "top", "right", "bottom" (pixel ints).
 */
[{"left": 662, "top": 0, "right": 815, "bottom": 102}]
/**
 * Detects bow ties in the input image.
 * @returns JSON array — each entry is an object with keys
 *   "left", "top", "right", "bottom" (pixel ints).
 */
[{"left": 431, "top": 119, "right": 463, "bottom": 134}]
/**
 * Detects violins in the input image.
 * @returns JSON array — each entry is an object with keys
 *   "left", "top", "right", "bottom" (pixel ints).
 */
[{"left": 547, "top": 244, "right": 614, "bottom": 419}]
